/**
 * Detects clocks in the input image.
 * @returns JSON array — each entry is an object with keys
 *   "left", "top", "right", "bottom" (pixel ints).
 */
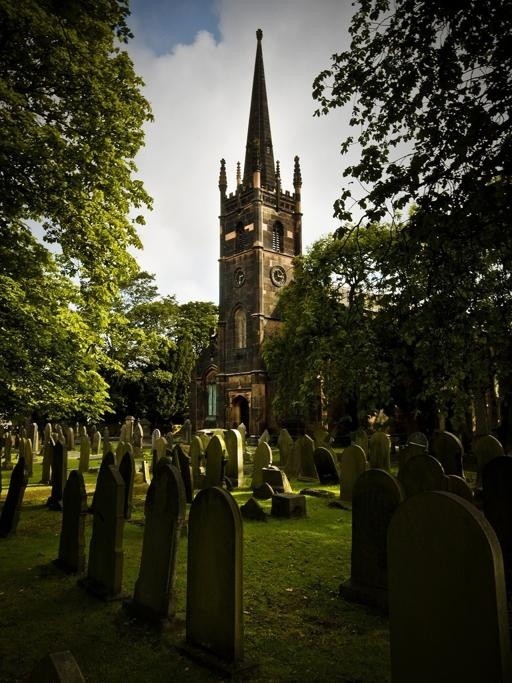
[
  {"left": 233, "top": 267, "right": 246, "bottom": 288},
  {"left": 271, "top": 265, "right": 287, "bottom": 287}
]
